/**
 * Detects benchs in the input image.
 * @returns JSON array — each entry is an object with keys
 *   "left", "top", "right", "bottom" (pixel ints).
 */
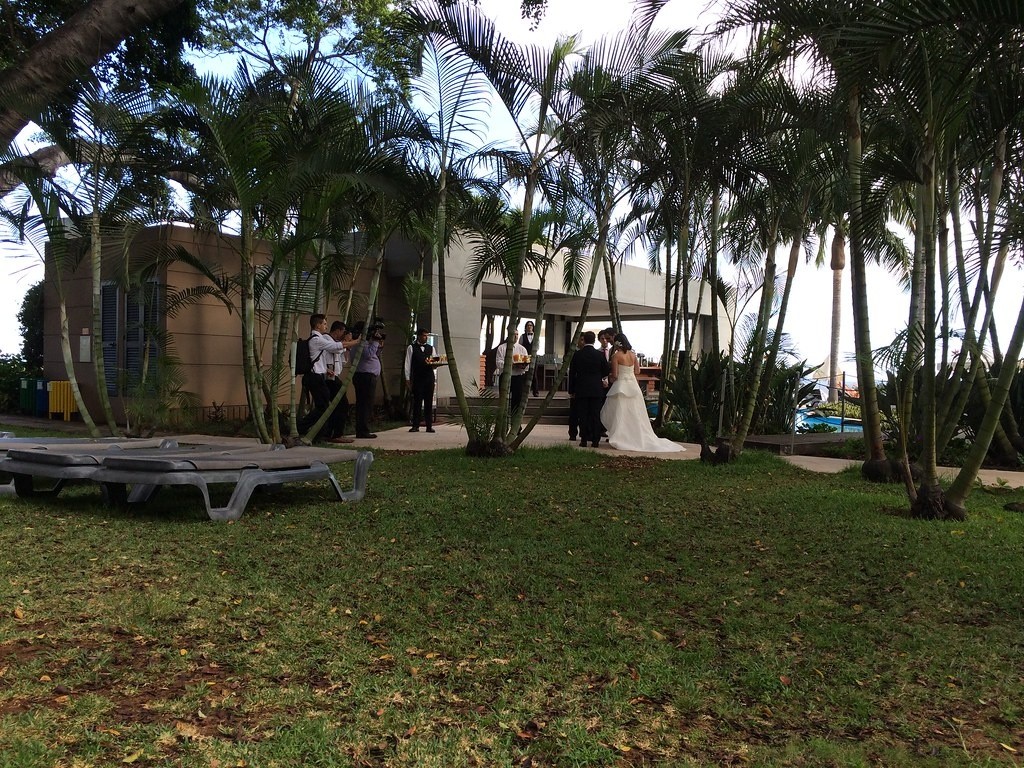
[{"left": 0, "top": 432, "right": 373, "bottom": 522}]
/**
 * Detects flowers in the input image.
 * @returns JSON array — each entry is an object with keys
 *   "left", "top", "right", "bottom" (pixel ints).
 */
[{"left": 615, "top": 341, "right": 622, "bottom": 347}]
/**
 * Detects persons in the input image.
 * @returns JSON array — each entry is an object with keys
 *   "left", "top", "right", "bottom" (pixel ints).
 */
[
  {"left": 495, "top": 329, "right": 530, "bottom": 437},
  {"left": 349, "top": 320, "right": 384, "bottom": 438},
  {"left": 600, "top": 333, "right": 687, "bottom": 453},
  {"left": 297, "top": 314, "right": 362, "bottom": 442},
  {"left": 568, "top": 332, "right": 585, "bottom": 442},
  {"left": 568, "top": 330, "right": 611, "bottom": 448},
  {"left": 519, "top": 321, "right": 540, "bottom": 397},
  {"left": 405, "top": 329, "right": 438, "bottom": 433},
  {"left": 602, "top": 327, "right": 618, "bottom": 443},
  {"left": 597, "top": 330, "right": 613, "bottom": 437}
]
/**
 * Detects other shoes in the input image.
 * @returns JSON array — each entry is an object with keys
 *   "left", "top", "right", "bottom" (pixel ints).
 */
[
  {"left": 426, "top": 427, "right": 435, "bottom": 432},
  {"left": 569, "top": 433, "right": 576, "bottom": 440},
  {"left": 320, "top": 435, "right": 332, "bottom": 442},
  {"left": 409, "top": 426, "right": 419, "bottom": 432},
  {"left": 533, "top": 391, "right": 539, "bottom": 397},
  {"left": 591, "top": 441, "right": 599, "bottom": 447},
  {"left": 332, "top": 437, "right": 354, "bottom": 443},
  {"left": 356, "top": 432, "right": 377, "bottom": 438},
  {"left": 600, "top": 432, "right": 607, "bottom": 437},
  {"left": 579, "top": 441, "right": 587, "bottom": 447}
]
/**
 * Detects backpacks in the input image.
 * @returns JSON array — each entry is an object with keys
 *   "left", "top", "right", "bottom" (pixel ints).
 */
[{"left": 288, "top": 334, "right": 324, "bottom": 374}]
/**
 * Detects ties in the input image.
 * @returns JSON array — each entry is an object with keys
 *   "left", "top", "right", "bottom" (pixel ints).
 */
[{"left": 602, "top": 348, "right": 607, "bottom": 357}]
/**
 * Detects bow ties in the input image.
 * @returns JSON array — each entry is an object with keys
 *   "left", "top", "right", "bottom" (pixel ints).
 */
[
  {"left": 419, "top": 344, "right": 426, "bottom": 348},
  {"left": 528, "top": 333, "right": 533, "bottom": 335}
]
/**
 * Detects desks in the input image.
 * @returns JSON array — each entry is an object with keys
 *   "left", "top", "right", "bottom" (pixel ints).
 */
[{"left": 539, "top": 363, "right": 569, "bottom": 391}]
